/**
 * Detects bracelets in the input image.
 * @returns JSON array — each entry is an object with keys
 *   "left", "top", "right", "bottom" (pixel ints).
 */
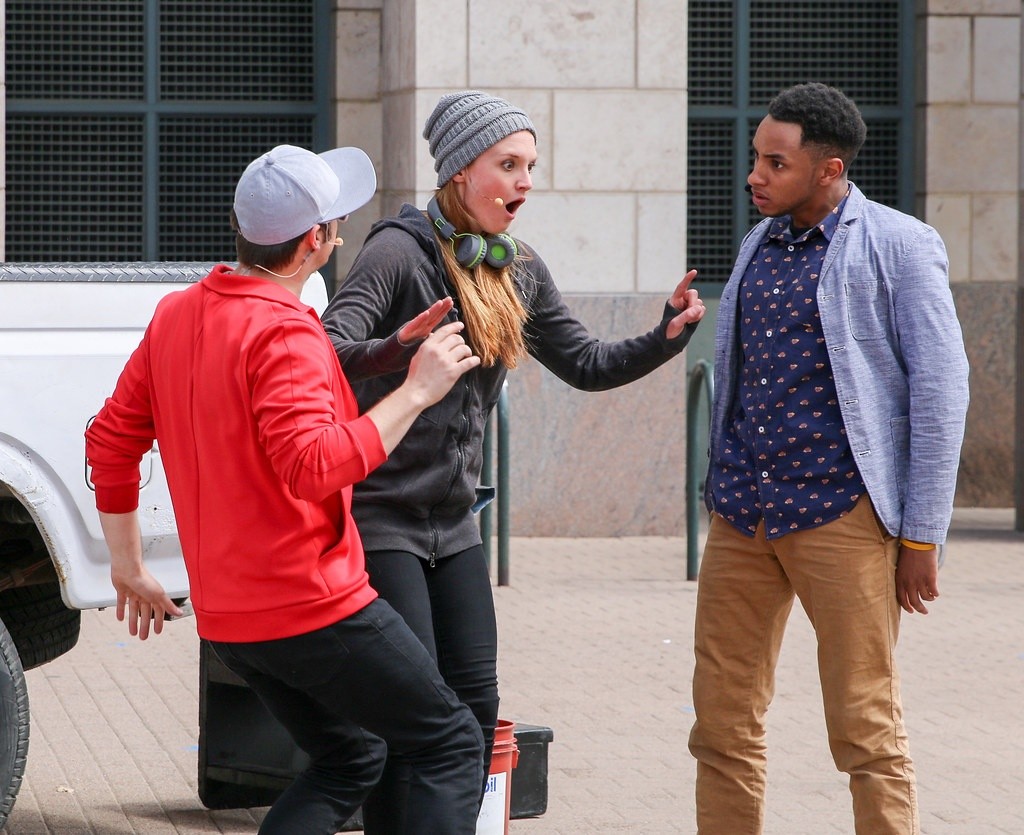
[{"left": 900, "top": 539, "right": 935, "bottom": 550}]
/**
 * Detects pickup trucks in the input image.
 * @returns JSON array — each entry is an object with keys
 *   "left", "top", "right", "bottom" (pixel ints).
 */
[{"left": 0, "top": 261, "right": 331, "bottom": 832}]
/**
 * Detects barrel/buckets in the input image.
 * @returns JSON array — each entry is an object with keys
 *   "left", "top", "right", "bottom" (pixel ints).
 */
[{"left": 474, "top": 720, "right": 519, "bottom": 835}]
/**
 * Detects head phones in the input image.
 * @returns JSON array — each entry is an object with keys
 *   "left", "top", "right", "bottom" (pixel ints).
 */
[{"left": 426, "top": 196, "right": 517, "bottom": 270}]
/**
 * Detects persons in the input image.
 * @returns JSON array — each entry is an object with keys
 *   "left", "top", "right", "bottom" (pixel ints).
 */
[
  {"left": 321, "top": 90, "right": 705, "bottom": 821},
  {"left": 83, "top": 144, "right": 485, "bottom": 835},
  {"left": 688, "top": 81, "right": 972, "bottom": 835}
]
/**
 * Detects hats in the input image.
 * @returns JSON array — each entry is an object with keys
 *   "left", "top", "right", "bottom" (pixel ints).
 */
[
  {"left": 233, "top": 143, "right": 378, "bottom": 246},
  {"left": 422, "top": 90, "right": 538, "bottom": 188}
]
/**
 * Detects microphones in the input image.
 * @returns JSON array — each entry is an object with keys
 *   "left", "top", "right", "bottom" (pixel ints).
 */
[
  {"left": 745, "top": 184, "right": 753, "bottom": 192},
  {"left": 326, "top": 223, "right": 343, "bottom": 247},
  {"left": 471, "top": 184, "right": 503, "bottom": 206}
]
